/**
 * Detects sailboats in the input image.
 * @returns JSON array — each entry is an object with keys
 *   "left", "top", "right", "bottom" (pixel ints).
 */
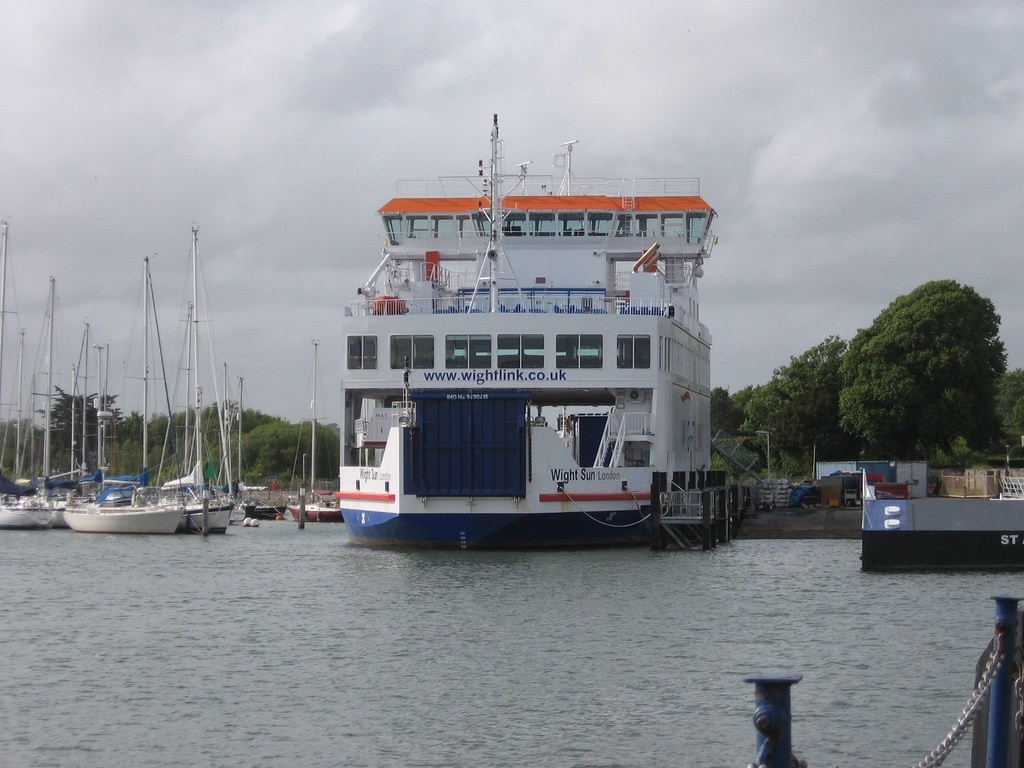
[{"left": 0, "top": 219, "right": 342, "bottom": 535}]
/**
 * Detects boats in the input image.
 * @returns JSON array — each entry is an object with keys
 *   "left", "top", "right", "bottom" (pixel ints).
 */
[
  {"left": 861, "top": 476, "right": 1024, "bottom": 574},
  {"left": 333, "top": 115, "right": 722, "bottom": 547}
]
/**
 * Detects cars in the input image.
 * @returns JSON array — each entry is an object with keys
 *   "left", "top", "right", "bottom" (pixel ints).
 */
[{"left": 788, "top": 470, "right": 862, "bottom": 510}]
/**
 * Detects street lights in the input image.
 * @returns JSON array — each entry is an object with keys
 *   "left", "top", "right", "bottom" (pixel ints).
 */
[{"left": 755, "top": 431, "right": 770, "bottom": 478}]
